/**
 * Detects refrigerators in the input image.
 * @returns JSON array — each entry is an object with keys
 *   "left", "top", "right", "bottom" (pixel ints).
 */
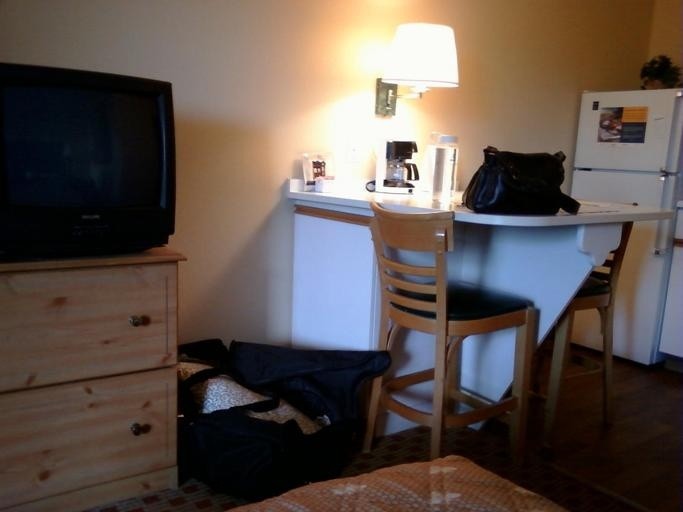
[{"left": 556, "top": 86, "right": 682, "bottom": 367}]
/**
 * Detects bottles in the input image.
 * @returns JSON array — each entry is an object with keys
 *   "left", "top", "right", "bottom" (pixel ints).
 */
[{"left": 430, "top": 134, "right": 461, "bottom": 205}]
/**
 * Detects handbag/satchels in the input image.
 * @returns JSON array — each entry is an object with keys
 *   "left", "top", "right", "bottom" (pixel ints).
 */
[{"left": 462, "top": 147, "right": 580, "bottom": 215}]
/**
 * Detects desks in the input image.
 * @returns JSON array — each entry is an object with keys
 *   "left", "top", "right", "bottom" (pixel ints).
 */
[{"left": 284, "top": 177, "right": 675, "bottom": 437}]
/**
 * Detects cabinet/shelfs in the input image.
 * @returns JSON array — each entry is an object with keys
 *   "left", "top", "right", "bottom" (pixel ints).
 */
[{"left": 1, "top": 247, "right": 191, "bottom": 512}]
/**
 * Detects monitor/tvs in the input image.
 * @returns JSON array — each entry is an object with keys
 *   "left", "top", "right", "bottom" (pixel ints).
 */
[{"left": 0, "top": 62, "right": 177, "bottom": 265}]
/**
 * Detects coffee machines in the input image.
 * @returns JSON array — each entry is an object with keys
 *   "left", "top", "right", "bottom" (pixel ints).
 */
[{"left": 375, "top": 137, "right": 420, "bottom": 195}]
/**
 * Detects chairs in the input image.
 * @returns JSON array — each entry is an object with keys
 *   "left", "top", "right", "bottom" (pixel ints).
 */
[
  {"left": 538, "top": 199, "right": 640, "bottom": 435},
  {"left": 359, "top": 199, "right": 542, "bottom": 462}
]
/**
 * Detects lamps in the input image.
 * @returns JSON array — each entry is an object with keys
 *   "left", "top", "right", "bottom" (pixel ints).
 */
[{"left": 372, "top": 20, "right": 460, "bottom": 122}]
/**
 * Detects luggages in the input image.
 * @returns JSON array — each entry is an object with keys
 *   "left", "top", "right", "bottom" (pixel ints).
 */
[{"left": 177, "top": 340, "right": 392, "bottom": 504}]
